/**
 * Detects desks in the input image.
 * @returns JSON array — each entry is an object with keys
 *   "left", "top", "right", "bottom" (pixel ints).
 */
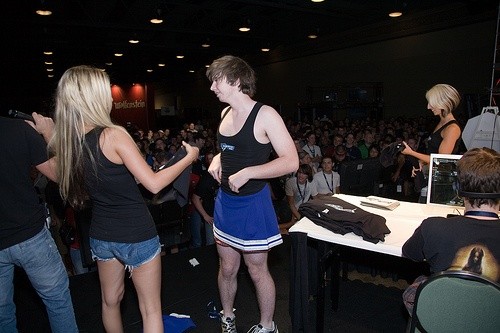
[{"left": 288, "top": 194, "right": 466, "bottom": 333}]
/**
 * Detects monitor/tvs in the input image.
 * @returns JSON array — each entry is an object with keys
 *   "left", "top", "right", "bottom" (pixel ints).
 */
[{"left": 427, "top": 153, "right": 466, "bottom": 210}]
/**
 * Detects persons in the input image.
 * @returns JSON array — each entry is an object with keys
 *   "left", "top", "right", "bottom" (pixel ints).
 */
[
  {"left": 47, "top": 65, "right": 201, "bottom": 333},
  {"left": 206, "top": 55, "right": 300, "bottom": 333},
  {"left": 0, "top": 111, "right": 79, "bottom": 333},
  {"left": 45, "top": 114, "right": 466, "bottom": 274},
  {"left": 400, "top": 84, "right": 462, "bottom": 204},
  {"left": 401, "top": 146, "right": 500, "bottom": 288}
]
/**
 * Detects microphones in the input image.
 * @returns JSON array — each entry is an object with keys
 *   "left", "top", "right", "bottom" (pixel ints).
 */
[{"left": 8, "top": 110, "right": 36, "bottom": 126}]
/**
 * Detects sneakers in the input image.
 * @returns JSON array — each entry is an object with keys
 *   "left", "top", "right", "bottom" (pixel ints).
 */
[{"left": 218, "top": 309, "right": 279, "bottom": 333}]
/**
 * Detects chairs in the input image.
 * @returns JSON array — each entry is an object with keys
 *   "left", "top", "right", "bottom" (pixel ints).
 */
[
  {"left": 148, "top": 201, "right": 192, "bottom": 255},
  {"left": 410, "top": 271, "right": 500, "bottom": 333}
]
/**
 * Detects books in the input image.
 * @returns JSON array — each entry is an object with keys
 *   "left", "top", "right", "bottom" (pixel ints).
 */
[{"left": 360, "top": 196, "right": 400, "bottom": 211}]
